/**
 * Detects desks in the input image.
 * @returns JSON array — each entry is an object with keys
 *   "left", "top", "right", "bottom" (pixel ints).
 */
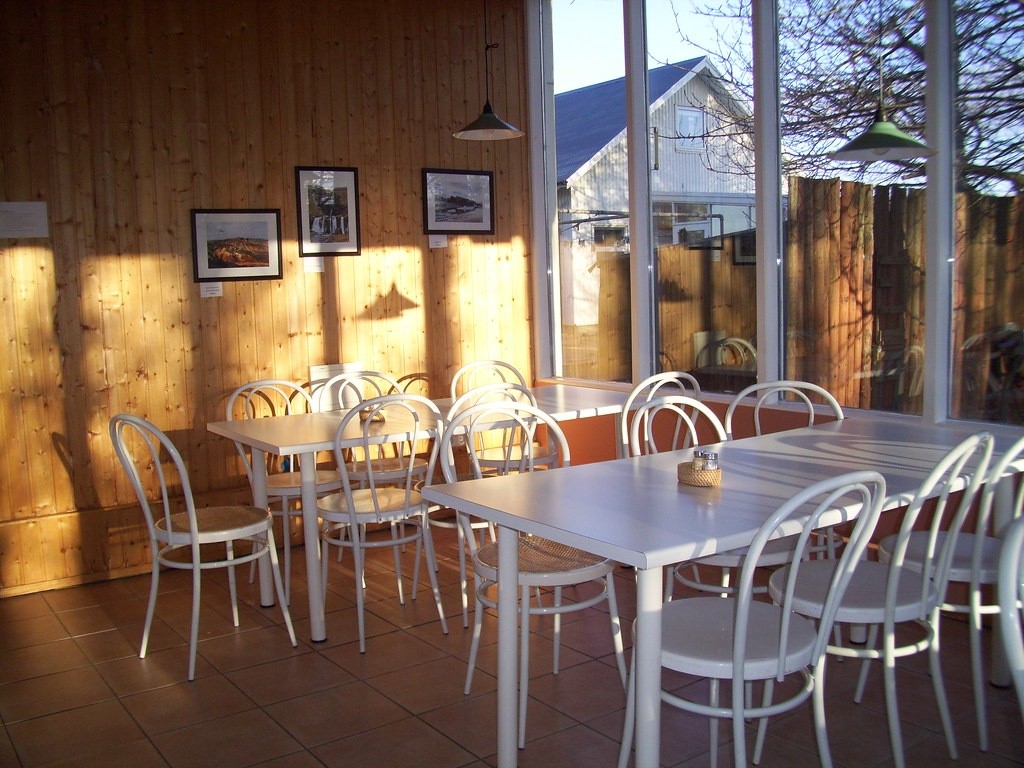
[
  {"left": 422, "top": 418, "right": 1024, "bottom": 768},
  {"left": 206, "top": 384, "right": 658, "bottom": 644}
]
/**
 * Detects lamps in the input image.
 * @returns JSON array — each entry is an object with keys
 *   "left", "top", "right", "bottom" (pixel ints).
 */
[
  {"left": 826, "top": 0, "right": 939, "bottom": 161},
  {"left": 453, "top": 0, "right": 525, "bottom": 140}
]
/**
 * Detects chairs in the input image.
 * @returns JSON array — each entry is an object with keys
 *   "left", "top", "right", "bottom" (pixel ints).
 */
[
  {"left": 226, "top": 359, "right": 845, "bottom": 696},
  {"left": 109, "top": 411, "right": 299, "bottom": 683},
  {"left": 617, "top": 430, "right": 1024, "bottom": 768}
]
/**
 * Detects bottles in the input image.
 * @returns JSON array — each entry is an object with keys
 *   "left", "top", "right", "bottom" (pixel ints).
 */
[
  {"left": 693, "top": 450, "right": 704, "bottom": 471},
  {"left": 703, "top": 453, "right": 718, "bottom": 470}
]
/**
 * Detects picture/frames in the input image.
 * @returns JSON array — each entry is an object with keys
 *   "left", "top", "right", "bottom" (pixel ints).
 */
[
  {"left": 422, "top": 167, "right": 495, "bottom": 235},
  {"left": 190, "top": 209, "right": 283, "bottom": 282},
  {"left": 295, "top": 166, "right": 361, "bottom": 257}
]
